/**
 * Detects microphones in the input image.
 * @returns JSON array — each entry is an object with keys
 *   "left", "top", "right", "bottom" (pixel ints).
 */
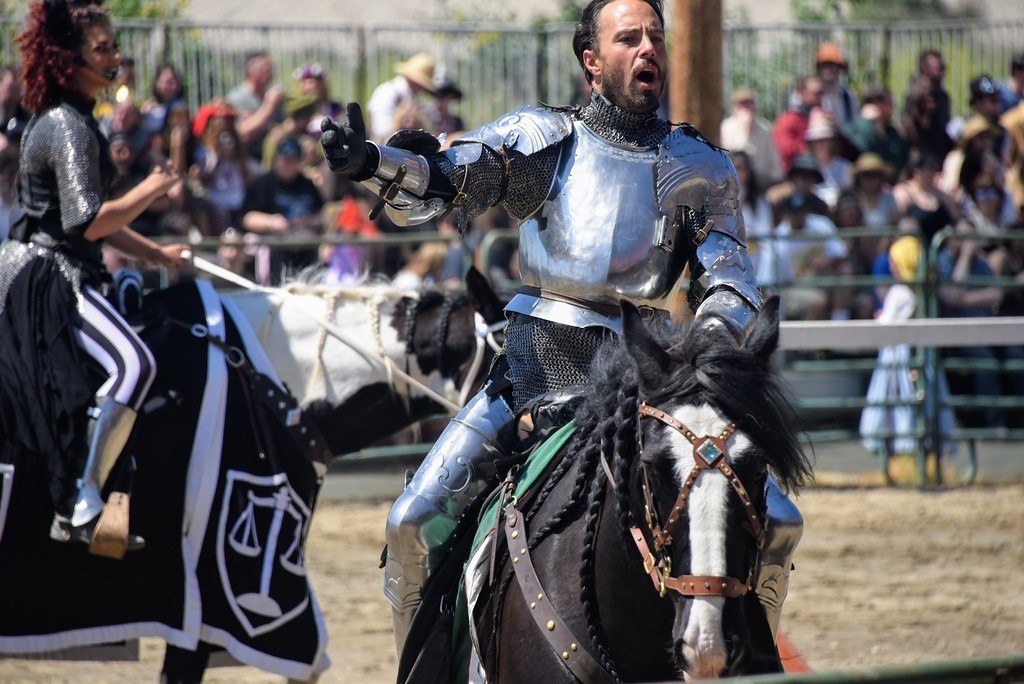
[{"left": 63, "top": 55, "right": 118, "bottom": 80}]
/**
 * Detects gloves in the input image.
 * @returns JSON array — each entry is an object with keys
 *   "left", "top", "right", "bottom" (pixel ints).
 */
[{"left": 321, "top": 102, "right": 380, "bottom": 181}]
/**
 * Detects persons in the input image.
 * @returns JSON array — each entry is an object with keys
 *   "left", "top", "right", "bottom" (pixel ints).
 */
[
  {"left": 1, "top": 39, "right": 1024, "bottom": 489},
  {"left": 9, "top": 0, "right": 200, "bottom": 552},
  {"left": 320, "top": 0, "right": 817, "bottom": 684}
]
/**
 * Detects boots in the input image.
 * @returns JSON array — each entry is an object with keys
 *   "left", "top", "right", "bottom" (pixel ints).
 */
[{"left": 50, "top": 394, "right": 144, "bottom": 552}]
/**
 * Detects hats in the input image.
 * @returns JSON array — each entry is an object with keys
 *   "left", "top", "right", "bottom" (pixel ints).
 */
[
  {"left": 957, "top": 116, "right": 1000, "bottom": 146},
  {"left": 276, "top": 137, "right": 302, "bottom": 159},
  {"left": 815, "top": 44, "right": 845, "bottom": 66},
  {"left": 787, "top": 155, "right": 825, "bottom": 184},
  {"left": 193, "top": 102, "right": 236, "bottom": 136},
  {"left": 968, "top": 73, "right": 1003, "bottom": 105},
  {"left": 732, "top": 87, "right": 757, "bottom": 102},
  {"left": 891, "top": 236, "right": 926, "bottom": 283},
  {"left": 394, "top": 53, "right": 436, "bottom": 93},
  {"left": 803, "top": 105, "right": 835, "bottom": 141},
  {"left": 852, "top": 153, "right": 892, "bottom": 175}
]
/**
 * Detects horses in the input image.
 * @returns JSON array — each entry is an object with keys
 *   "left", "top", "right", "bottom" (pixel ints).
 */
[{"left": 0, "top": 243, "right": 810, "bottom": 684}]
